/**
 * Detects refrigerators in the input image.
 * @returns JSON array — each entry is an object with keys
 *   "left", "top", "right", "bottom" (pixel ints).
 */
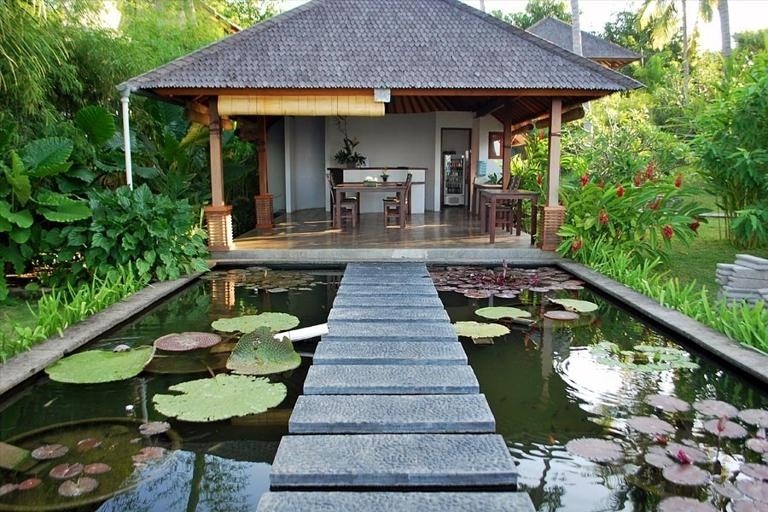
[{"left": 444, "top": 155, "right": 465, "bottom": 207}]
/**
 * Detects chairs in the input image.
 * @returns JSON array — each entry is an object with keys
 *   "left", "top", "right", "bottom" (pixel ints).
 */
[
  {"left": 485, "top": 173, "right": 520, "bottom": 234},
  {"left": 383, "top": 173, "right": 413, "bottom": 226},
  {"left": 326, "top": 173, "right": 358, "bottom": 226}
]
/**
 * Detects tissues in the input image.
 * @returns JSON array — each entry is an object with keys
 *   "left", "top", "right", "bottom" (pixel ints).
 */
[{"left": 362, "top": 176, "right": 378, "bottom": 187}]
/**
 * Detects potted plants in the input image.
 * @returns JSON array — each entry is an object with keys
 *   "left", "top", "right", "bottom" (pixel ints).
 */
[{"left": 333, "top": 136, "right": 368, "bottom": 168}]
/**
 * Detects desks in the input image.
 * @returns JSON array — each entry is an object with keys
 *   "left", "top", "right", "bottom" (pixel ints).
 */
[
  {"left": 480, "top": 189, "right": 541, "bottom": 245},
  {"left": 332, "top": 182, "right": 408, "bottom": 229},
  {"left": 473, "top": 183, "right": 502, "bottom": 220}
]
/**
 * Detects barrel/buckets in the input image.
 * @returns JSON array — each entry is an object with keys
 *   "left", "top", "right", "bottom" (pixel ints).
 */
[{"left": 475, "top": 159, "right": 487, "bottom": 176}]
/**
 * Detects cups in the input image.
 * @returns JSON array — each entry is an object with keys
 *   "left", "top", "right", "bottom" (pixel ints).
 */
[{"left": 397, "top": 183, "right": 402, "bottom": 186}]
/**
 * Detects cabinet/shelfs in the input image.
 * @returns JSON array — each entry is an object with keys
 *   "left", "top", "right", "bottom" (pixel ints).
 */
[{"left": 326, "top": 167, "right": 428, "bottom": 215}]
